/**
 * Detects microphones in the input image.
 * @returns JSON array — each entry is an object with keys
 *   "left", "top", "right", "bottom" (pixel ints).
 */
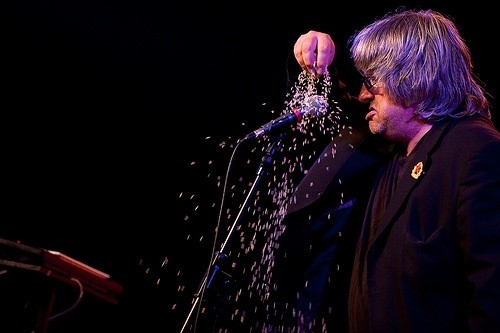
[{"left": 239, "top": 94, "right": 327, "bottom": 144}]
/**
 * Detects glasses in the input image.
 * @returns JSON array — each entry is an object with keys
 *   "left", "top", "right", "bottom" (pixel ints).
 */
[{"left": 361, "top": 74, "right": 379, "bottom": 88}]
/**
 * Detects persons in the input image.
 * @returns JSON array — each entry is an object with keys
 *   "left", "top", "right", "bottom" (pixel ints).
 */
[{"left": 292, "top": 9, "right": 500, "bottom": 333}]
